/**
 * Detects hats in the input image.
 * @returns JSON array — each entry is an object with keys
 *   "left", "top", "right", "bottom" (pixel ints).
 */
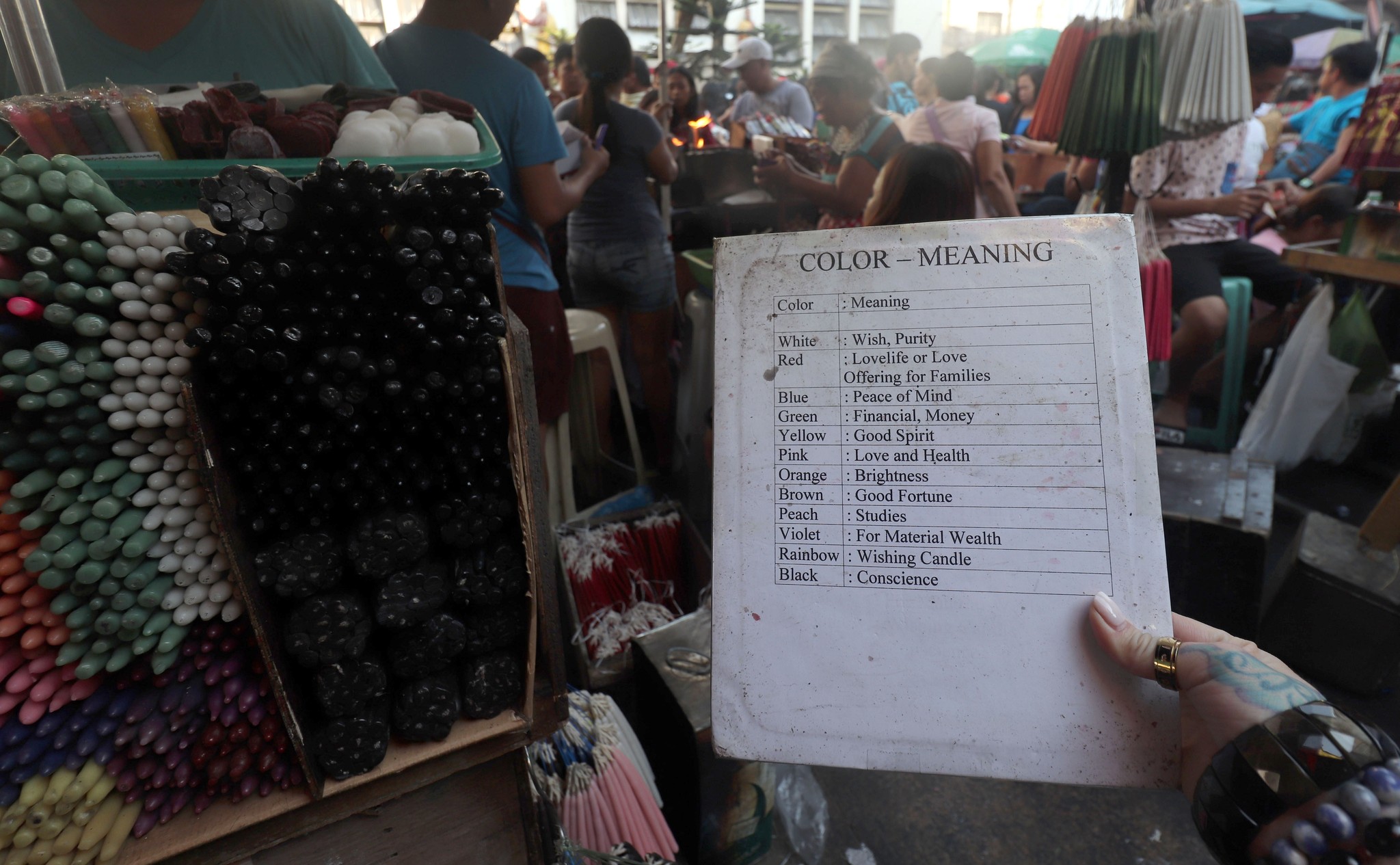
[
  {"left": 807, "top": 39, "right": 881, "bottom": 93},
  {"left": 720, "top": 37, "right": 773, "bottom": 69}
]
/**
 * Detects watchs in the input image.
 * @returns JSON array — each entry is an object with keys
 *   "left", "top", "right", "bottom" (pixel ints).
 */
[{"left": 1298, "top": 176, "right": 1315, "bottom": 190}]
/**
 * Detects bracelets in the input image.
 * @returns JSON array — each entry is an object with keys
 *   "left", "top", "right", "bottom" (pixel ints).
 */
[
  {"left": 1023, "top": 138, "right": 1033, "bottom": 152},
  {"left": 1192, "top": 699, "right": 1400, "bottom": 865}
]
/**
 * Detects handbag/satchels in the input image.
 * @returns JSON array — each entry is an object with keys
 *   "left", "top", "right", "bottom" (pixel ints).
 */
[{"left": 1232, "top": 285, "right": 1398, "bottom": 473}]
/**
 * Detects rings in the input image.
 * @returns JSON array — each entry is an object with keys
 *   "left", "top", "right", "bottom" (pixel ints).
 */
[{"left": 1148, "top": 633, "right": 1185, "bottom": 694}]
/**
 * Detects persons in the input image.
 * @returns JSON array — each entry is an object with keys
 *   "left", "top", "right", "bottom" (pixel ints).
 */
[
  {"left": 505, "top": 42, "right": 748, "bottom": 149},
  {"left": 748, "top": 41, "right": 906, "bottom": 229},
  {"left": 1126, "top": 28, "right": 1335, "bottom": 432},
  {"left": 906, "top": 50, "right": 1021, "bottom": 218},
  {"left": 552, "top": 16, "right": 697, "bottom": 484},
  {"left": 1246, "top": 40, "right": 1379, "bottom": 232},
  {"left": 1086, "top": 589, "right": 1400, "bottom": 864},
  {"left": 875, "top": 31, "right": 1103, "bottom": 195},
  {"left": 820, "top": 140, "right": 979, "bottom": 228},
  {"left": 714, "top": 37, "right": 816, "bottom": 138},
  {"left": 367, "top": 0, "right": 609, "bottom": 450}
]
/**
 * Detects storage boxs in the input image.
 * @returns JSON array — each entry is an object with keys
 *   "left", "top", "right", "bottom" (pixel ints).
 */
[{"left": 556, "top": 507, "right": 711, "bottom": 692}]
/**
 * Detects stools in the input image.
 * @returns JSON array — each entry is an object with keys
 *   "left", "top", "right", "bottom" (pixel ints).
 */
[
  {"left": 678, "top": 295, "right": 718, "bottom": 440},
  {"left": 544, "top": 311, "right": 646, "bottom": 528},
  {"left": 1149, "top": 276, "right": 1254, "bottom": 454}
]
